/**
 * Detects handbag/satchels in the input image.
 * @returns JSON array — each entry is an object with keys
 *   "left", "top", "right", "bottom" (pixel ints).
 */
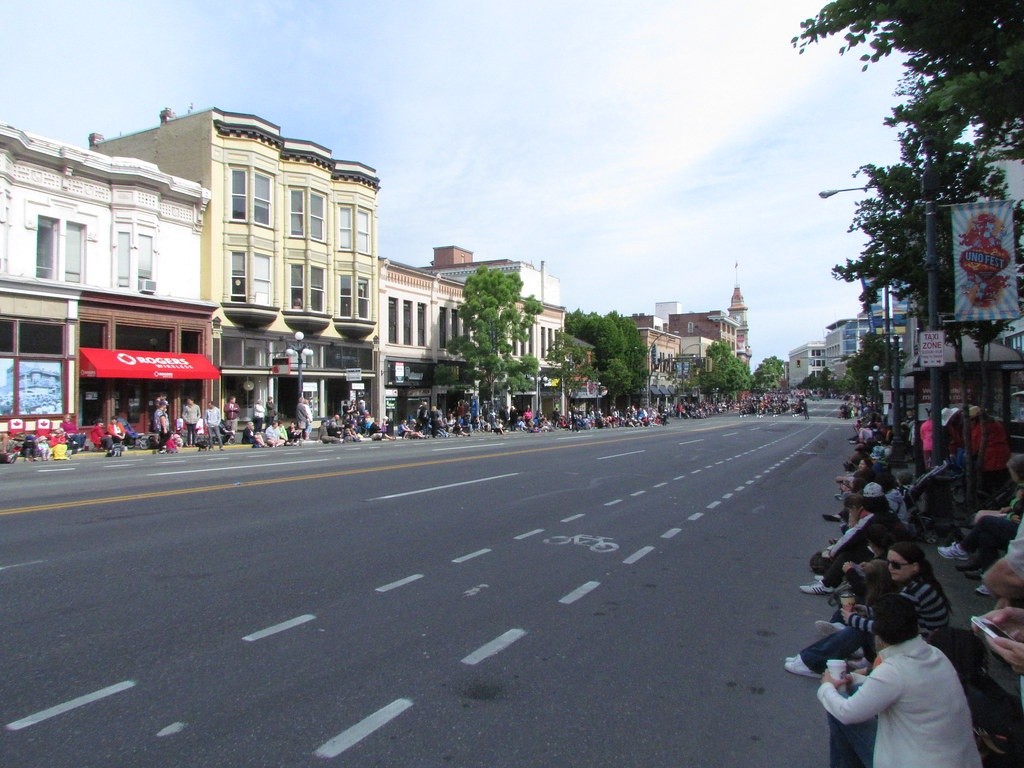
[{"left": 827, "top": 581, "right": 853, "bottom": 607}]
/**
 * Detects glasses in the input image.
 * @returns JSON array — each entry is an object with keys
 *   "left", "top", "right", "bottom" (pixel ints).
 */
[{"left": 887, "top": 559, "right": 911, "bottom": 570}]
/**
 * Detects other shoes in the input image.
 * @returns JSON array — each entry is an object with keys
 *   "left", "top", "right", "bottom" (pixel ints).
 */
[
  {"left": 955, "top": 561, "right": 981, "bottom": 571},
  {"left": 835, "top": 494, "right": 843, "bottom": 500},
  {"left": 976, "top": 584, "right": 990, "bottom": 595},
  {"left": 823, "top": 514, "right": 841, "bottom": 522},
  {"left": 974, "top": 727, "right": 1007, "bottom": 754},
  {"left": 815, "top": 621, "right": 847, "bottom": 637},
  {"left": 965, "top": 571, "right": 982, "bottom": 579}
]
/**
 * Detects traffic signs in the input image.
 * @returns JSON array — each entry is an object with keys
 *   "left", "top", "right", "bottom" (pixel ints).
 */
[{"left": 919, "top": 331, "right": 946, "bottom": 368}]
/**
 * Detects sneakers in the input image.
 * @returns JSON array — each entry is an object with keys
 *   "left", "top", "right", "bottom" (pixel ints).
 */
[
  {"left": 800, "top": 580, "right": 834, "bottom": 595},
  {"left": 783, "top": 654, "right": 822, "bottom": 678},
  {"left": 938, "top": 541, "right": 969, "bottom": 560},
  {"left": 814, "top": 575, "right": 824, "bottom": 580},
  {"left": 847, "top": 658, "right": 873, "bottom": 671}
]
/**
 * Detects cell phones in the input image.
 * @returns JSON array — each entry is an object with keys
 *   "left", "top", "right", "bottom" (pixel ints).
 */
[{"left": 971, "top": 616, "right": 1014, "bottom": 641}]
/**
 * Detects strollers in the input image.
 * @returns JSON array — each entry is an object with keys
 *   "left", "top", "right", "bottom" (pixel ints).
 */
[
  {"left": 886, "top": 459, "right": 949, "bottom": 545},
  {"left": 117, "top": 417, "right": 151, "bottom": 450},
  {"left": 209, "top": 419, "right": 237, "bottom": 445}
]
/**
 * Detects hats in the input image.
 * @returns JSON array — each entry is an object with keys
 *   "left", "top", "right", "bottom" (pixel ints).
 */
[
  {"left": 969, "top": 406, "right": 980, "bottom": 415},
  {"left": 870, "top": 446, "right": 885, "bottom": 459},
  {"left": 159, "top": 401, "right": 170, "bottom": 405},
  {"left": 862, "top": 482, "right": 884, "bottom": 497},
  {"left": 38, "top": 436, "right": 48, "bottom": 442},
  {"left": 941, "top": 407, "right": 960, "bottom": 426}
]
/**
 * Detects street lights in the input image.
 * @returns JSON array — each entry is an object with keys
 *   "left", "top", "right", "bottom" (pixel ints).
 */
[
  {"left": 818, "top": 186, "right": 893, "bottom": 425},
  {"left": 285, "top": 330, "right": 314, "bottom": 397},
  {"left": 529, "top": 366, "right": 548, "bottom": 418},
  {"left": 592, "top": 381, "right": 602, "bottom": 419},
  {"left": 868, "top": 366, "right": 885, "bottom": 411},
  {"left": 647, "top": 330, "right": 680, "bottom": 407},
  {"left": 681, "top": 342, "right": 703, "bottom": 394}
]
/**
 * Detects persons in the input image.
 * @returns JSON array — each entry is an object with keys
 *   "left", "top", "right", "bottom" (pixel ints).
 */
[
  {"left": 669, "top": 389, "right": 812, "bottom": 421},
  {"left": 784, "top": 547, "right": 1024, "bottom": 768},
  {"left": 821, "top": 394, "right": 1024, "bottom": 573},
  {"left": 242, "top": 396, "right": 666, "bottom": 450},
  {"left": 0, "top": 393, "right": 240, "bottom": 464},
  {"left": 815, "top": 388, "right": 853, "bottom": 402}
]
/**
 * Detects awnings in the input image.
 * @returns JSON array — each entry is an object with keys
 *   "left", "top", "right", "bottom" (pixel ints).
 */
[
  {"left": 903, "top": 329, "right": 1024, "bottom": 378},
  {"left": 649, "top": 385, "right": 676, "bottom": 397},
  {"left": 78, "top": 346, "right": 221, "bottom": 380}
]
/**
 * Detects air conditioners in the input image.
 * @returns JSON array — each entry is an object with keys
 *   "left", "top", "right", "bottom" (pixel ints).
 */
[{"left": 138, "top": 279, "right": 156, "bottom": 295}]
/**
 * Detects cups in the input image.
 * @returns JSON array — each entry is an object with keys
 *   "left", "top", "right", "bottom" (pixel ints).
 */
[
  {"left": 839, "top": 592, "right": 857, "bottom": 613},
  {"left": 826, "top": 659, "right": 846, "bottom": 695}
]
